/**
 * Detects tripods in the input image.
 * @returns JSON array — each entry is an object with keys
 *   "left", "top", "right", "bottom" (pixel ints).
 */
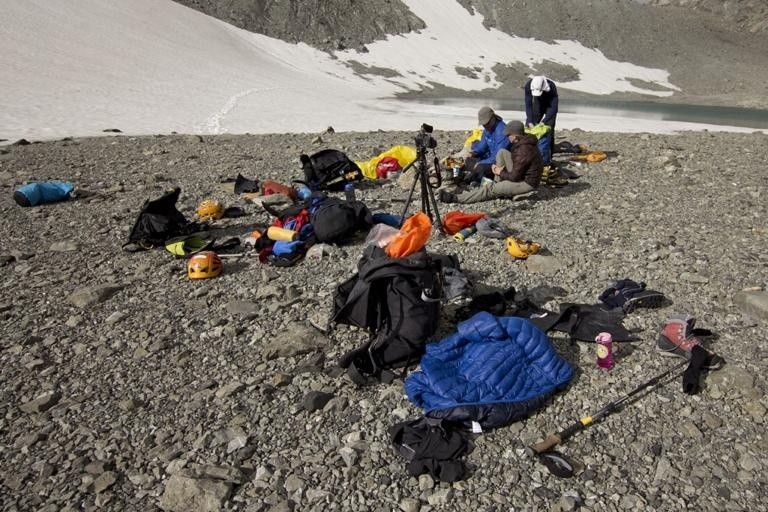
[{"left": 398, "top": 153, "right": 442, "bottom": 230}]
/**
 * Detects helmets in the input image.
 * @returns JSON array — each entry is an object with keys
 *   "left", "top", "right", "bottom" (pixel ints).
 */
[
  {"left": 506, "top": 235, "right": 540, "bottom": 258},
  {"left": 187, "top": 250, "right": 224, "bottom": 279},
  {"left": 197, "top": 198, "right": 225, "bottom": 220}
]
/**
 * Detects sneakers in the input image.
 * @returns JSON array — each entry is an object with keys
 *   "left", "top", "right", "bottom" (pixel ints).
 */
[{"left": 439, "top": 189, "right": 458, "bottom": 203}]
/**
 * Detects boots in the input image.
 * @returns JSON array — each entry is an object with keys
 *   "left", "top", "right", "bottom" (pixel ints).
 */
[
  {"left": 620, "top": 278, "right": 665, "bottom": 313},
  {"left": 656, "top": 313, "right": 721, "bottom": 369}
]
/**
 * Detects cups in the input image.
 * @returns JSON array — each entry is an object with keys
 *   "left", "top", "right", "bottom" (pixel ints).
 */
[{"left": 596, "top": 333, "right": 614, "bottom": 367}]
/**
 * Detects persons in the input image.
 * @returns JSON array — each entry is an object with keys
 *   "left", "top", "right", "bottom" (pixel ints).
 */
[
  {"left": 441, "top": 106, "right": 511, "bottom": 188},
  {"left": 523, "top": 75, "right": 559, "bottom": 167},
  {"left": 438, "top": 119, "right": 543, "bottom": 204}
]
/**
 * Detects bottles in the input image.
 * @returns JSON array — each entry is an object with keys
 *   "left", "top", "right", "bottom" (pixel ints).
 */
[
  {"left": 453, "top": 164, "right": 459, "bottom": 177},
  {"left": 346, "top": 183, "right": 355, "bottom": 203},
  {"left": 268, "top": 225, "right": 301, "bottom": 242}
]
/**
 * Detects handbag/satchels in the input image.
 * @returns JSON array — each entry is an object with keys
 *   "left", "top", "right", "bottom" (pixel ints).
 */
[
  {"left": 310, "top": 198, "right": 368, "bottom": 242},
  {"left": 330, "top": 244, "right": 442, "bottom": 373}
]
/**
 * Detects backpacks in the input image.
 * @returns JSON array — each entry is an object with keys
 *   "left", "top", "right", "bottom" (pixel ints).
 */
[{"left": 298, "top": 149, "right": 364, "bottom": 192}]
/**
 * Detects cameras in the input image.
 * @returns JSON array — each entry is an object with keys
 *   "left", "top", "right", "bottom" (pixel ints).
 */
[{"left": 414, "top": 123, "right": 437, "bottom": 149}]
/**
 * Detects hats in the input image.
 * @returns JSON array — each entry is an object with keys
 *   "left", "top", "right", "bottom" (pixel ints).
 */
[
  {"left": 501, "top": 120, "right": 524, "bottom": 136},
  {"left": 479, "top": 106, "right": 493, "bottom": 126},
  {"left": 529, "top": 75, "right": 551, "bottom": 97}
]
